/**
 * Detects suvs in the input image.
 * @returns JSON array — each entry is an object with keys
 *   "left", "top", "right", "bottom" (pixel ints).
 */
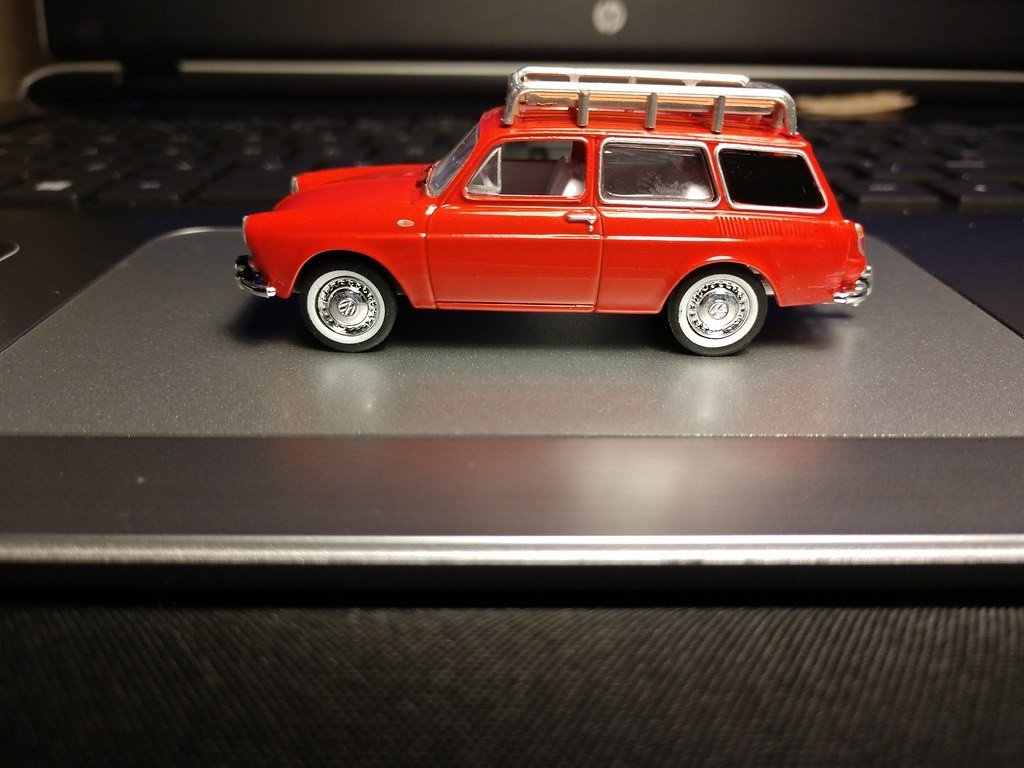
[{"left": 233, "top": 65, "right": 872, "bottom": 357}]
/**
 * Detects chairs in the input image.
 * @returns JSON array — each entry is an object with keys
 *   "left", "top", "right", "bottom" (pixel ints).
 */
[
  {"left": 544, "top": 156, "right": 572, "bottom": 195},
  {"left": 553, "top": 169, "right": 583, "bottom": 196}
]
